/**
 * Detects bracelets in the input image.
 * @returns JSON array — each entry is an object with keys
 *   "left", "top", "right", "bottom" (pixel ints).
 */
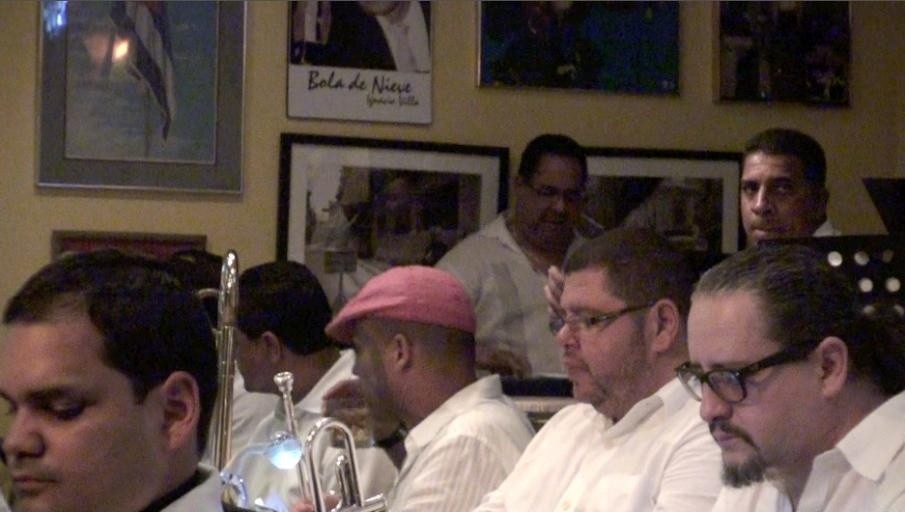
[{"left": 371, "top": 421, "right": 408, "bottom": 450}]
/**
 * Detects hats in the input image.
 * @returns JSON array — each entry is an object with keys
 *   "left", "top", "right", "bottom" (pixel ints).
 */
[{"left": 324, "top": 265, "right": 475, "bottom": 343}]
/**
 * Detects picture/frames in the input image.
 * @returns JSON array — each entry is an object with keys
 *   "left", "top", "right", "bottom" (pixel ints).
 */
[
  {"left": 277, "top": 131, "right": 510, "bottom": 319},
  {"left": 33, "top": 1, "right": 246, "bottom": 198},
  {"left": 546, "top": 147, "right": 745, "bottom": 270},
  {"left": 50, "top": 226, "right": 207, "bottom": 269}
]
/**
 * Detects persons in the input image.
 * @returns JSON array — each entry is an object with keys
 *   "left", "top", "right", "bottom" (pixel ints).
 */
[
  {"left": 403, "top": 176, "right": 474, "bottom": 264},
  {"left": 1, "top": 246, "right": 229, "bottom": 511},
  {"left": 684, "top": 248, "right": 904, "bottom": 511},
  {"left": 434, "top": 133, "right": 609, "bottom": 378},
  {"left": 463, "top": 228, "right": 795, "bottom": 511},
  {"left": 169, "top": 250, "right": 281, "bottom": 475},
  {"left": 218, "top": 258, "right": 401, "bottom": 512},
  {"left": 320, "top": 263, "right": 537, "bottom": 512},
  {"left": 740, "top": 125, "right": 828, "bottom": 244},
  {"left": 319, "top": 2, "right": 430, "bottom": 75},
  {"left": 385, "top": 172, "right": 429, "bottom": 266}
]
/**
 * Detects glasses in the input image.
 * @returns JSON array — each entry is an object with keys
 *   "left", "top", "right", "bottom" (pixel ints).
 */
[
  {"left": 523, "top": 178, "right": 586, "bottom": 204},
  {"left": 675, "top": 344, "right": 803, "bottom": 403},
  {"left": 549, "top": 305, "right": 649, "bottom": 335}
]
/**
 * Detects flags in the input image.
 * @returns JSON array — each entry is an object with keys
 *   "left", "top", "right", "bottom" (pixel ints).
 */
[{"left": 109, "top": 1, "right": 174, "bottom": 142}]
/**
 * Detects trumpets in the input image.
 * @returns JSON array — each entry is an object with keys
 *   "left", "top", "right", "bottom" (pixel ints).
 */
[{"left": 273, "top": 370, "right": 388, "bottom": 510}]
